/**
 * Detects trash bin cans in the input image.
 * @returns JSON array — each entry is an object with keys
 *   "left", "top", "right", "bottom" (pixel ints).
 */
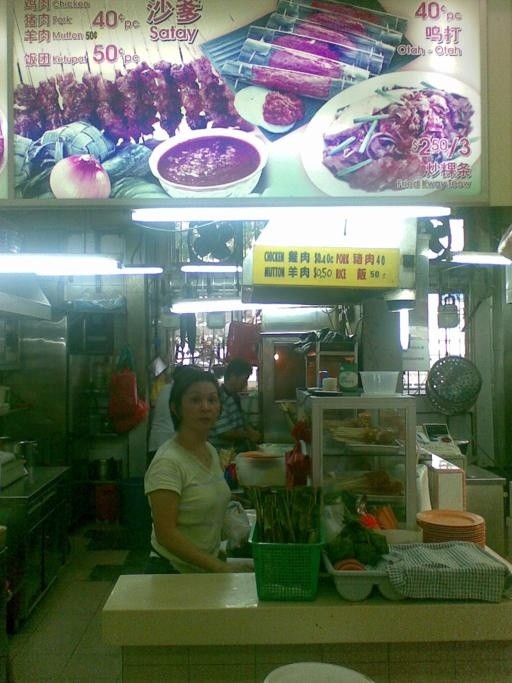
[{"left": 93, "top": 483, "right": 123, "bottom": 523}]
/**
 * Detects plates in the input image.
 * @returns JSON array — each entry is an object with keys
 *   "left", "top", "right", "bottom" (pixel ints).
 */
[
  {"left": 297, "top": 70, "right": 481, "bottom": 196},
  {"left": 415, "top": 508, "right": 487, "bottom": 551}
]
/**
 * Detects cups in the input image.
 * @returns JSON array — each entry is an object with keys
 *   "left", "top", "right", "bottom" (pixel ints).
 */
[{"left": 322, "top": 377, "right": 337, "bottom": 391}]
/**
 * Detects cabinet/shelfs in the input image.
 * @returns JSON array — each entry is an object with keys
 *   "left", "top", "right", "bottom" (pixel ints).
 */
[
  {"left": 293, "top": 340, "right": 419, "bottom": 552},
  {"left": 59, "top": 280, "right": 131, "bottom": 480},
  {"left": 0, "top": 467, "right": 75, "bottom": 635},
  {"left": 0, "top": 361, "right": 31, "bottom": 420}
]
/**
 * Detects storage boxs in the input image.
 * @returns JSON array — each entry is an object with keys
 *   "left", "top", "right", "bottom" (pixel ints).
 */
[{"left": 232, "top": 454, "right": 287, "bottom": 488}]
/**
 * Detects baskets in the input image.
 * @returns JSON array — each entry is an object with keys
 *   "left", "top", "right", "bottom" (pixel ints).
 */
[{"left": 248, "top": 485, "right": 325, "bottom": 602}]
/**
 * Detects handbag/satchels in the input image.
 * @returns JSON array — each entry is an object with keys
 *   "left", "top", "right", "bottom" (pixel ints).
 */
[{"left": 109, "top": 345, "right": 148, "bottom": 433}]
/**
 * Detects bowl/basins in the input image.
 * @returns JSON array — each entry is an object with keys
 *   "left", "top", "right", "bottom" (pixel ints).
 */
[{"left": 147, "top": 126, "right": 270, "bottom": 198}]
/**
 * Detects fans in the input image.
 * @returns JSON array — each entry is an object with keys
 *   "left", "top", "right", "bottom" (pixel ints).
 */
[{"left": 425, "top": 356, "right": 483, "bottom": 448}]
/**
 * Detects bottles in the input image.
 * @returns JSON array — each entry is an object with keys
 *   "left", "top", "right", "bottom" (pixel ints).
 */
[
  {"left": 93, "top": 355, "right": 111, "bottom": 385},
  {"left": 318, "top": 368, "right": 329, "bottom": 388},
  {"left": 96, "top": 455, "right": 113, "bottom": 480},
  {"left": 336, "top": 355, "right": 361, "bottom": 396}
]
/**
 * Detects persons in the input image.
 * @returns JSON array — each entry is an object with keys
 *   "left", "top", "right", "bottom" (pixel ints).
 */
[
  {"left": 144, "top": 368, "right": 252, "bottom": 574},
  {"left": 206, "top": 358, "right": 261, "bottom": 454},
  {"left": 147, "top": 365, "right": 200, "bottom": 469}
]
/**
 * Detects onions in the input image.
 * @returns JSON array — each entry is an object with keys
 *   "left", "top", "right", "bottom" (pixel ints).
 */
[{"left": 49, "top": 154, "right": 111, "bottom": 199}]
